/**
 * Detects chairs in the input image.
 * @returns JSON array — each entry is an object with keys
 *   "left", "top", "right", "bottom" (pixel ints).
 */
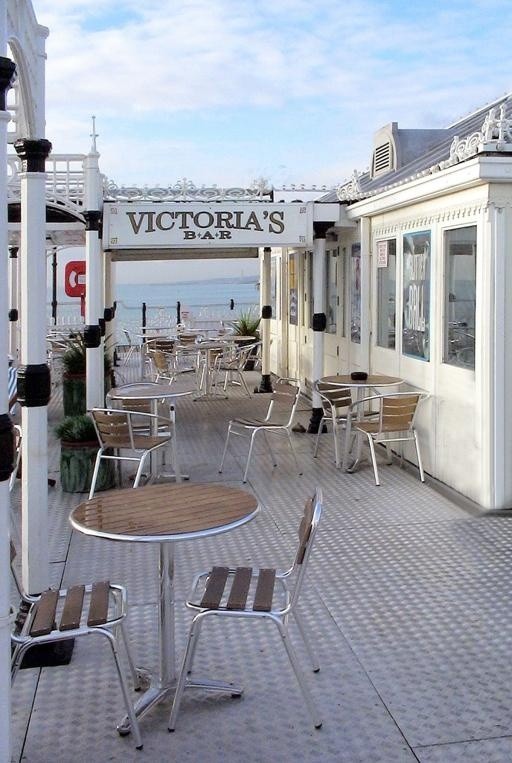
[
  {"left": 343, "top": 389, "right": 430, "bottom": 486},
  {"left": 217, "top": 376, "right": 305, "bottom": 482},
  {"left": 113, "top": 322, "right": 262, "bottom": 404},
  {"left": 83, "top": 405, "right": 175, "bottom": 498},
  {"left": 311, "top": 377, "right": 394, "bottom": 470},
  {"left": 163, "top": 486, "right": 331, "bottom": 734},
  {"left": 9, "top": 536, "right": 147, "bottom": 752},
  {"left": 448, "top": 321, "right": 475, "bottom": 368},
  {"left": 104, "top": 380, "right": 172, "bottom": 467}
]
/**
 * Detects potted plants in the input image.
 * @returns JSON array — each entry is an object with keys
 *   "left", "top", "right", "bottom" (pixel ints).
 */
[{"left": 226, "top": 305, "right": 263, "bottom": 371}]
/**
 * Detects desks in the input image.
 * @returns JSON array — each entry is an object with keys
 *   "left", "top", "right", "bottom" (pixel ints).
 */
[
  {"left": 318, "top": 371, "right": 408, "bottom": 474},
  {"left": 69, "top": 477, "right": 263, "bottom": 738},
  {"left": 112, "top": 381, "right": 191, "bottom": 486}
]
